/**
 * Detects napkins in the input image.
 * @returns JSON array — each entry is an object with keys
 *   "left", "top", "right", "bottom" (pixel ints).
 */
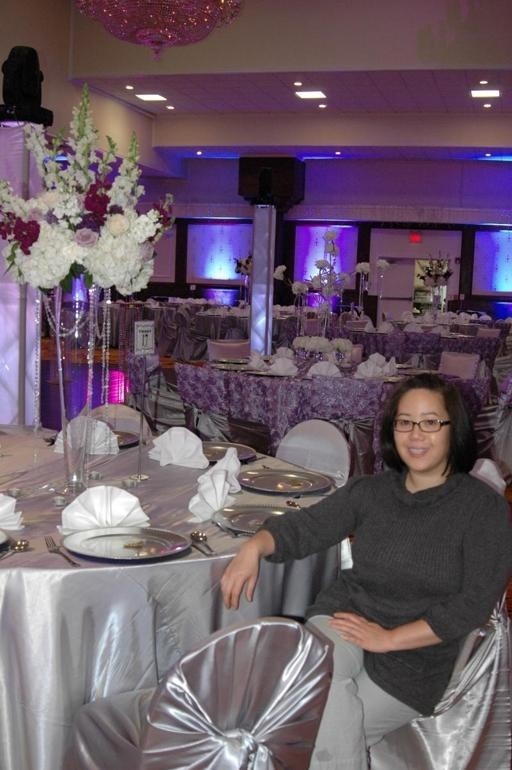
[
  {"left": 60, "top": 486, "right": 153, "bottom": 531},
  {"left": 184, "top": 471, "right": 242, "bottom": 521},
  {"left": 0, "top": 491, "right": 30, "bottom": 531}
]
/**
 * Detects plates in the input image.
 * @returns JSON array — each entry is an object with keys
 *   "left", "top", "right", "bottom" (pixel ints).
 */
[
  {"left": 60, "top": 525, "right": 192, "bottom": 560},
  {"left": 390, "top": 320, "right": 480, "bottom": 339},
  {"left": 212, "top": 357, "right": 440, "bottom": 382},
  {"left": 110, "top": 431, "right": 138, "bottom": 447},
  {"left": 237, "top": 469, "right": 332, "bottom": 492},
  {"left": 1, "top": 527, "right": 10, "bottom": 546},
  {"left": 202, "top": 441, "right": 256, "bottom": 462},
  {"left": 214, "top": 504, "right": 301, "bottom": 534}
]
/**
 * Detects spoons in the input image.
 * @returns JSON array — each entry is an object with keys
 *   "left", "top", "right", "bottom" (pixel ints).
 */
[
  {"left": 190, "top": 528, "right": 215, "bottom": 554},
  {"left": 285, "top": 500, "right": 301, "bottom": 508},
  {"left": 1, "top": 539, "right": 29, "bottom": 562}
]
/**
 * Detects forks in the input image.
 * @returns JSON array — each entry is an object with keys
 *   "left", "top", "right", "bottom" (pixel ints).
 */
[{"left": 43, "top": 535, "right": 79, "bottom": 566}]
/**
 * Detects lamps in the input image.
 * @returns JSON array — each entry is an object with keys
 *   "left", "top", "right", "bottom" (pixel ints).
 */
[{"left": 73, "top": 0, "right": 241, "bottom": 62}]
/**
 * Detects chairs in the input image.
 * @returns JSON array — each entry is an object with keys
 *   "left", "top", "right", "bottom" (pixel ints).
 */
[
  {"left": 365, "top": 616, "right": 511, "bottom": 769},
  {"left": 65, "top": 616, "right": 330, "bottom": 770}
]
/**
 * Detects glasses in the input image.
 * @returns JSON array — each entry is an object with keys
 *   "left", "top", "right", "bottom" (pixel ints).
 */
[{"left": 392, "top": 418, "right": 449, "bottom": 433}]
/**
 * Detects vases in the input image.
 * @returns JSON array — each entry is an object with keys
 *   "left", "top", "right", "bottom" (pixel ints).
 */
[
  {"left": 54, "top": 270, "right": 100, "bottom": 498},
  {"left": 432, "top": 285, "right": 440, "bottom": 325},
  {"left": 240, "top": 274, "right": 249, "bottom": 308}
]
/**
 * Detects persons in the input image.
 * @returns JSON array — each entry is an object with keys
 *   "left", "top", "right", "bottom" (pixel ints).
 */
[{"left": 219, "top": 373, "right": 512, "bottom": 770}]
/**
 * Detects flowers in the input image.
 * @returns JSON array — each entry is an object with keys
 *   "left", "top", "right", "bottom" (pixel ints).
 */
[
  {"left": 414, "top": 248, "right": 455, "bottom": 287},
  {"left": 375, "top": 259, "right": 389, "bottom": 272},
  {"left": 355, "top": 262, "right": 372, "bottom": 274},
  {"left": 231, "top": 253, "right": 253, "bottom": 277},
  {"left": 273, "top": 264, "right": 309, "bottom": 295},
  {"left": 310, "top": 231, "right": 349, "bottom": 297},
  {"left": 0, "top": 78, "right": 176, "bottom": 299}
]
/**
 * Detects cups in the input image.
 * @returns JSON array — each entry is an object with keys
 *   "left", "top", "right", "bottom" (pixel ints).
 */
[
  {"left": 33, "top": 442, "right": 55, "bottom": 492},
  {"left": 58, "top": 408, "right": 93, "bottom": 501}
]
